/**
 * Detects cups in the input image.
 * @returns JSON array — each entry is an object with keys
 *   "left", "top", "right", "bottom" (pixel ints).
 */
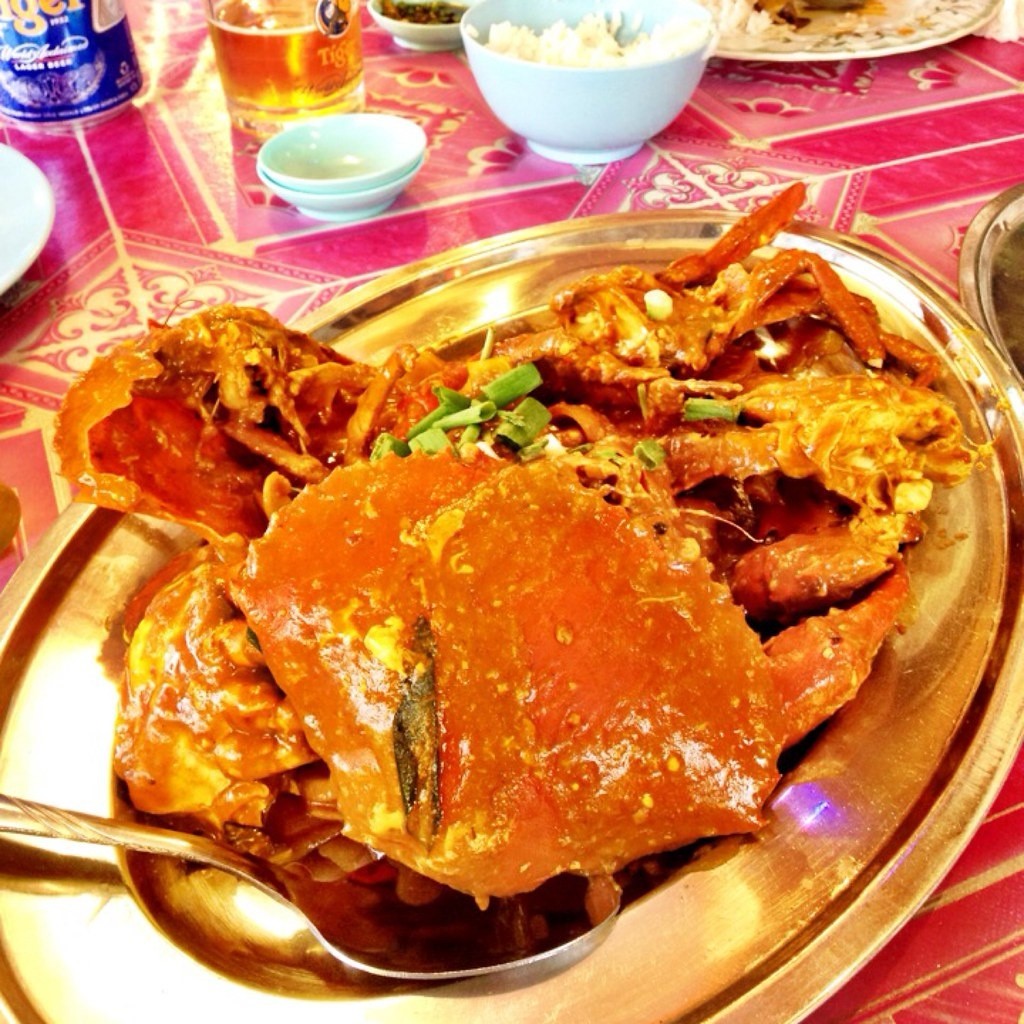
[{"left": 207, "top": 1, "right": 369, "bottom": 139}]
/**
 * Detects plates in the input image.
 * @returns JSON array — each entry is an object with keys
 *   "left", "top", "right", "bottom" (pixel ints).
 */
[
  {"left": 0, "top": 208, "right": 1023, "bottom": 1022},
  {"left": 0, "top": 142, "right": 55, "bottom": 296},
  {"left": 705, "top": 0, "right": 1000, "bottom": 65}
]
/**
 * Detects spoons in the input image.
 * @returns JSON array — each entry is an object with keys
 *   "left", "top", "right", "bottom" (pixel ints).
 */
[{"left": 0, "top": 795, "right": 624, "bottom": 985}]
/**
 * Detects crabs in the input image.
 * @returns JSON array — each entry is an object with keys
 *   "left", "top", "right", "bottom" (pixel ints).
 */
[{"left": 54, "top": 182, "right": 989, "bottom": 933}]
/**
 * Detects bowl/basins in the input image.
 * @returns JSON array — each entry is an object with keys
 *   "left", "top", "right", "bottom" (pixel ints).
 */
[
  {"left": 459, "top": 2, "right": 728, "bottom": 174},
  {"left": 365, "top": 0, "right": 463, "bottom": 50},
  {"left": 252, "top": 110, "right": 426, "bottom": 220}
]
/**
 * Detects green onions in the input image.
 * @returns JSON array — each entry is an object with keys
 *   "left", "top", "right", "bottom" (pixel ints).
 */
[{"left": 367, "top": 362, "right": 738, "bottom": 463}]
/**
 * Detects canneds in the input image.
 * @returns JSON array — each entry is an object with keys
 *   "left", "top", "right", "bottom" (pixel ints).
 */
[{"left": 1, "top": 0, "right": 146, "bottom": 137}]
